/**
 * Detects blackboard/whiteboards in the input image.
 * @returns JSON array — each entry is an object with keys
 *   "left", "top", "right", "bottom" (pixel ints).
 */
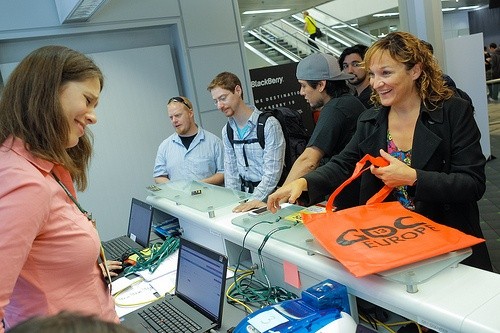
[{"left": 0, "top": 45, "right": 203, "bottom": 244}]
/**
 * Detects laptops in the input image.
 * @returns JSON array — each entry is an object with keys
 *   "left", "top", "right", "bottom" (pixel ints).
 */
[
  {"left": 118, "top": 236, "right": 229, "bottom": 333},
  {"left": 97, "top": 196, "right": 153, "bottom": 265}
]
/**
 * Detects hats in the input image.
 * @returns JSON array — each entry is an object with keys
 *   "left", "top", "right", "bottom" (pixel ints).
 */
[{"left": 296, "top": 54, "right": 355, "bottom": 80}]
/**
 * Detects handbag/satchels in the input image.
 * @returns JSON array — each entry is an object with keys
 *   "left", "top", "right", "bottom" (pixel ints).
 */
[
  {"left": 300, "top": 154, "right": 485, "bottom": 278},
  {"left": 307, "top": 17, "right": 321, "bottom": 38}
]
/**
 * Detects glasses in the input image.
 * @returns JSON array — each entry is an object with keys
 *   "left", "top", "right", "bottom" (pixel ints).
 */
[{"left": 167, "top": 97, "right": 191, "bottom": 109}]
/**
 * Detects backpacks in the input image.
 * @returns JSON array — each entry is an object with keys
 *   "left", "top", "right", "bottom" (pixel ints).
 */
[{"left": 227, "top": 107, "right": 311, "bottom": 193}]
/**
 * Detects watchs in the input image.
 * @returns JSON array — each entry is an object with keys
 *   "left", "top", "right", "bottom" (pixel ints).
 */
[{"left": 411, "top": 178, "right": 417, "bottom": 186}]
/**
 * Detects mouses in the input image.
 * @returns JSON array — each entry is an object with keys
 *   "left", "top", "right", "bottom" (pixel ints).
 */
[{"left": 109, "top": 265, "right": 124, "bottom": 278}]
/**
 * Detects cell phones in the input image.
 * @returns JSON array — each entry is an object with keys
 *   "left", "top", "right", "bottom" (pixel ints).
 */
[{"left": 249, "top": 205, "right": 268, "bottom": 215}]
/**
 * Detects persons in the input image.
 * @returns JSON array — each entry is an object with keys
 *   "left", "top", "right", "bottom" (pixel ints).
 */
[
  {"left": 302, "top": 11, "right": 319, "bottom": 54},
  {"left": 4, "top": 313, "right": 136, "bottom": 333},
  {"left": 207, "top": 71, "right": 286, "bottom": 203},
  {"left": 98, "top": 259, "right": 123, "bottom": 279},
  {"left": 310, "top": 100, "right": 324, "bottom": 127},
  {"left": 484, "top": 45, "right": 494, "bottom": 99},
  {"left": 0, "top": 46, "right": 122, "bottom": 333},
  {"left": 153, "top": 95, "right": 225, "bottom": 186},
  {"left": 486, "top": 42, "right": 500, "bottom": 102},
  {"left": 267, "top": 32, "right": 494, "bottom": 274},
  {"left": 419, "top": 40, "right": 456, "bottom": 94},
  {"left": 231, "top": 52, "right": 368, "bottom": 214},
  {"left": 337, "top": 44, "right": 380, "bottom": 110}
]
